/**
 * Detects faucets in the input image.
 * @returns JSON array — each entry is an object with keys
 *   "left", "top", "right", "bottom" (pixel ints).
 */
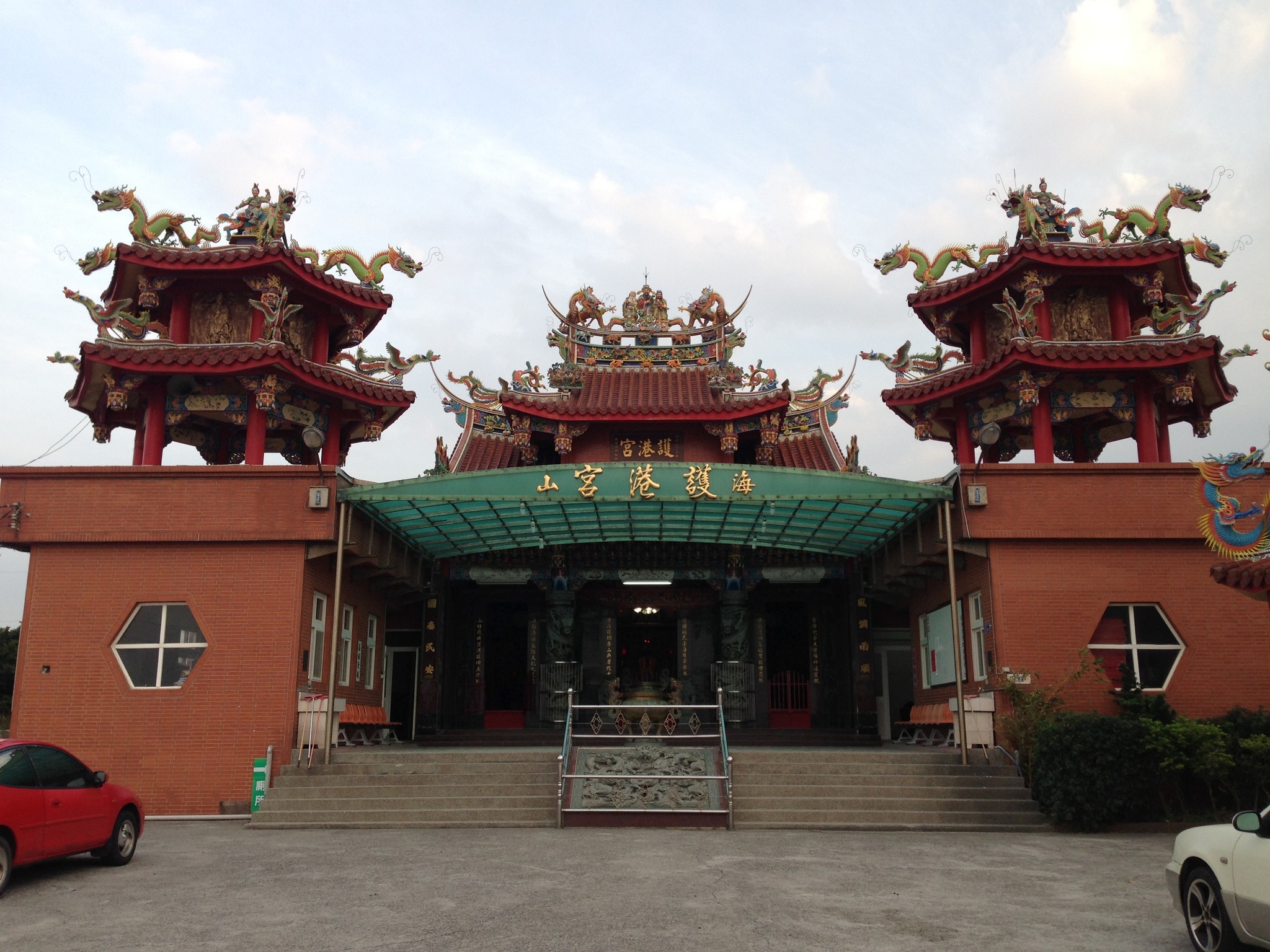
[
  {"left": 308, "top": 688, "right": 316, "bottom": 691},
  {"left": 977, "top": 687, "right": 982, "bottom": 692}
]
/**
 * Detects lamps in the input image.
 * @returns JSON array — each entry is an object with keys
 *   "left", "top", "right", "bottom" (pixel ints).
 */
[
  {"left": 619, "top": 567, "right": 675, "bottom": 584},
  {"left": 974, "top": 421, "right": 1001, "bottom": 476},
  {"left": 303, "top": 426, "right": 326, "bottom": 486}
]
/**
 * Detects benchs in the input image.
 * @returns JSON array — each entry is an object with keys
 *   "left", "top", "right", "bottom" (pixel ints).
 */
[
  {"left": 337, "top": 704, "right": 403, "bottom": 745},
  {"left": 894, "top": 704, "right": 954, "bottom": 747}
]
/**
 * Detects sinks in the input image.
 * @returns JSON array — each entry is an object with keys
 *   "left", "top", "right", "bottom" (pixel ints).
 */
[
  {"left": 949, "top": 691, "right": 995, "bottom": 712},
  {"left": 297, "top": 691, "right": 347, "bottom": 712}
]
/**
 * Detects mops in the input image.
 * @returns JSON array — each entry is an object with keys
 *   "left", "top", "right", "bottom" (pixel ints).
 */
[
  {"left": 296, "top": 694, "right": 329, "bottom": 769},
  {"left": 963, "top": 695, "right": 991, "bottom": 765}
]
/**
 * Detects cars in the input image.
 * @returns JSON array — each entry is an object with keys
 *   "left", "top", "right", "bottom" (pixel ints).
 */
[
  {"left": 1164, "top": 808, "right": 1270, "bottom": 951},
  {"left": 1, "top": 739, "right": 145, "bottom": 897}
]
